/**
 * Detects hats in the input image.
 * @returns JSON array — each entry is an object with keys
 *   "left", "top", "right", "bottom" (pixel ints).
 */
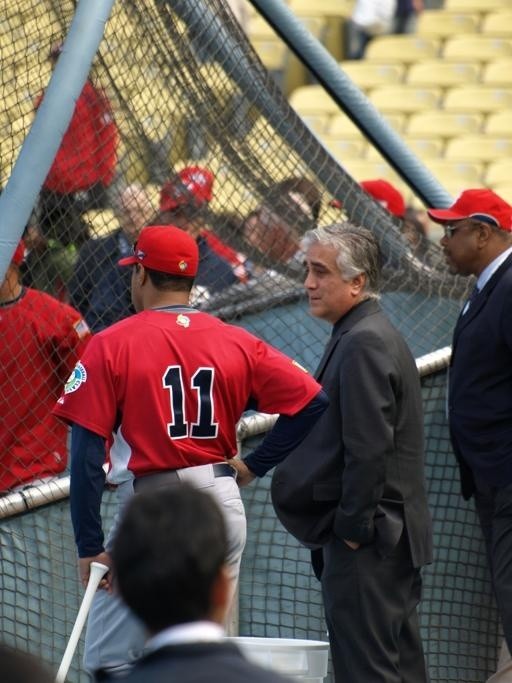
[
  {"left": 331, "top": 180, "right": 407, "bottom": 220},
  {"left": 11, "top": 239, "right": 27, "bottom": 267},
  {"left": 159, "top": 166, "right": 214, "bottom": 212},
  {"left": 118, "top": 225, "right": 199, "bottom": 280},
  {"left": 427, "top": 189, "right": 512, "bottom": 233}
]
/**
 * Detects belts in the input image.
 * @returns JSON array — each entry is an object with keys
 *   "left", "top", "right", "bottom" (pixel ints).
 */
[{"left": 132, "top": 464, "right": 237, "bottom": 492}]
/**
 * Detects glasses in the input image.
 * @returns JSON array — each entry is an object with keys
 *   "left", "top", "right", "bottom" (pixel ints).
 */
[{"left": 445, "top": 223, "right": 476, "bottom": 235}]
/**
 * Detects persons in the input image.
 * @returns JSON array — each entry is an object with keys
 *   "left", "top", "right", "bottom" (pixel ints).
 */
[
  {"left": 0, "top": 239, "right": 117, "bottom": 500},
  {"left": 270, "top": 222, "right": 435, "bottom": 683},
  {"left": 426, "top": 188, "right": 512, "bottom": 658},
  {"left": 34, "top": 42, "right": 118, "bottom": 246},
  {"left": 50, "top": 224, "right": 329, "bottom": 683},
  {"left": 96, "top": 480, "right": 302, "bottom": 681}
]
